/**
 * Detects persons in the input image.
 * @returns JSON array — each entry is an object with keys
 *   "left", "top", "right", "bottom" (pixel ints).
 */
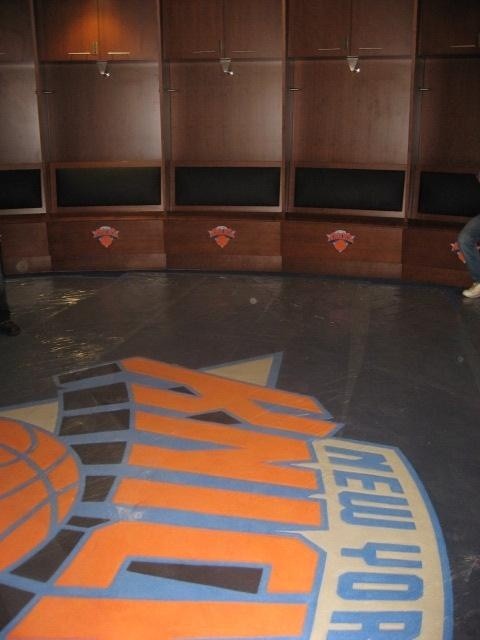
[
  {"left": 458, "top": 214, "right": 480, "bottom": 299},
  {"left": 1, "top": 243, "right": 21, "bottom": 336}
]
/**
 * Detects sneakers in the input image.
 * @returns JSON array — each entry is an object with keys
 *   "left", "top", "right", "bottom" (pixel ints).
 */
[{"left": 462, "top": 282, "right": 480, "bottom": 298}]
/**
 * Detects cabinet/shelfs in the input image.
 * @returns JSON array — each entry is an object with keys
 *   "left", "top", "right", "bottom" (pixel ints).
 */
[
  {"left": 38, "top": 1, "right": 167, "bottom": 278},
  {"left": 404, "top": 0, "right": 479, "bottom": 285},
  {"left": 0, "top": 2, "right": 53, "bottom": 281},
  {"left": 161, "top": 2, "right": 284, "bottom": 273},
  {"left": 287, "top": 0, "right": 415, "bottom": 282}
]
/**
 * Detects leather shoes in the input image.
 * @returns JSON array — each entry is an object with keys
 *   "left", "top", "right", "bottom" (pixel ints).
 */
[{"left": 0, "top": 320, "right": 21, "bottom": 337}]
